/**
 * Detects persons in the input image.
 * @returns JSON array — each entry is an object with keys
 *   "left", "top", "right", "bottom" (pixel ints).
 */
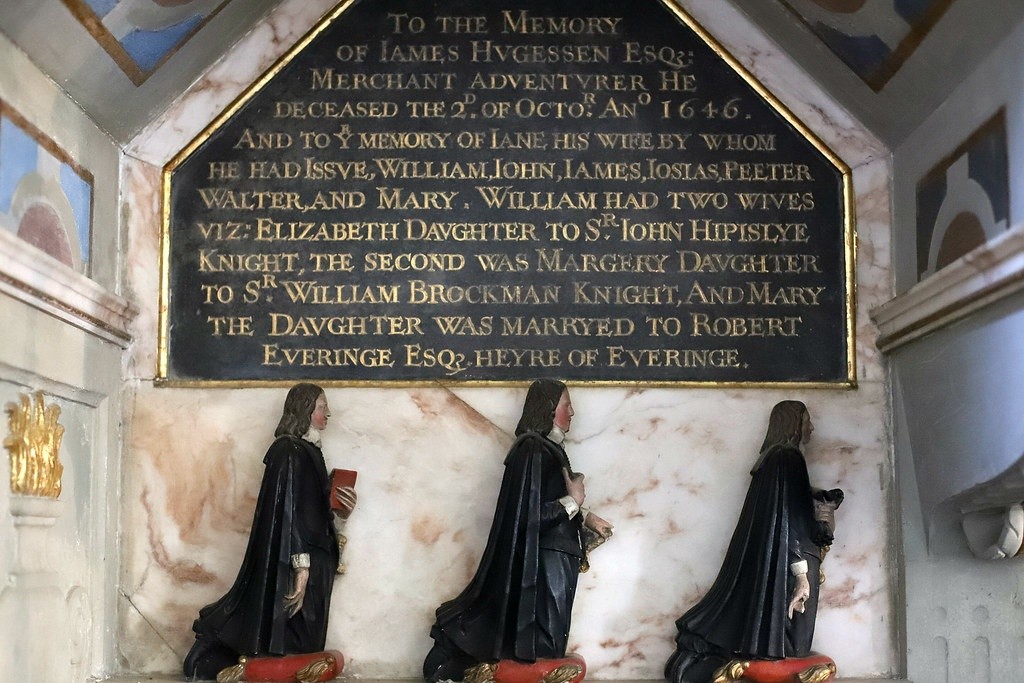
[
  {"left": 663, "top": 400, "right": 831, "bottom": 683},
  {"left": 422, "top": 377, "right": 613, "bottom": 682},
  {"left": 183, "top": 383, "right": 357, "bottom": 676}
]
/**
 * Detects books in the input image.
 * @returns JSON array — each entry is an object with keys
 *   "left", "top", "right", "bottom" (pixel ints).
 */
[{"left": 327, "top": 468, "right": 358, "bottom": 512}]
